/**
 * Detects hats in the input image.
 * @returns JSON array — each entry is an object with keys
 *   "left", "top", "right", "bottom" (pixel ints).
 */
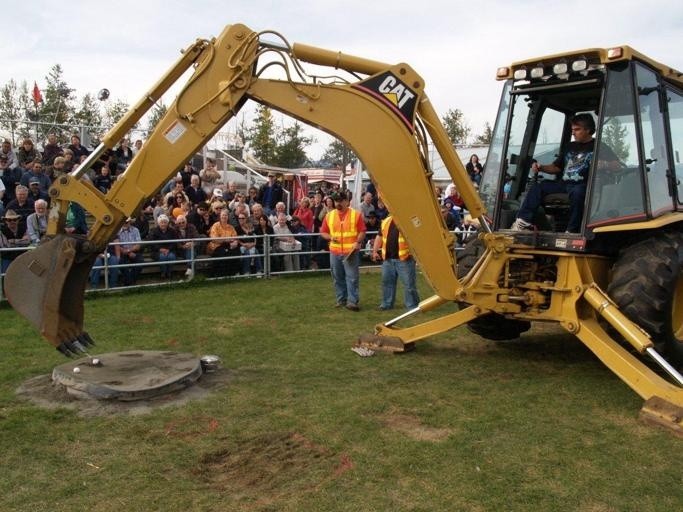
[
  {"left": 333, "top": 192, "right": 347, "bottom": 200},
  {"left": 213, "top": 189, "right": 223, "bottom": 197},
  {"left": 2, "top": 210, "right": 21, "bottom": 221},
  {"left": 268, "top": 171, "right": 276, "bottom": 177},
  {"left": 28, "top": 177, "right": 40, "bottom": 185}
]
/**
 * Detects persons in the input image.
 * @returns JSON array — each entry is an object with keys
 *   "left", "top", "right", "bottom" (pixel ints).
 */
[
  {"left": 508, "top": 109, "right": 625, "bottom": 235},
  {"left": 369, "top": 213, "right": 417, "bottom": 310},
  {"left": 0, "top": 124, "right": 481, "bottom": 293},
  {"left": 317, "top": 190, "right": 362, "bottom": 312}
]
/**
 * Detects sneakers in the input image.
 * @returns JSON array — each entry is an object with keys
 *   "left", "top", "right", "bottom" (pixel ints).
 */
[
  {"left": 335, "top": 303, "right": 359, "bottom": 312},
  {"left": 511, "top": 218, "right": 531, "bottom": 230}
]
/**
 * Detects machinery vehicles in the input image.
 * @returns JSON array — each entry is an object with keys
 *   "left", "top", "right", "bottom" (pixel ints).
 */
[{"left": 0, "top": 33, "right": 681, "bottom": 450}]
